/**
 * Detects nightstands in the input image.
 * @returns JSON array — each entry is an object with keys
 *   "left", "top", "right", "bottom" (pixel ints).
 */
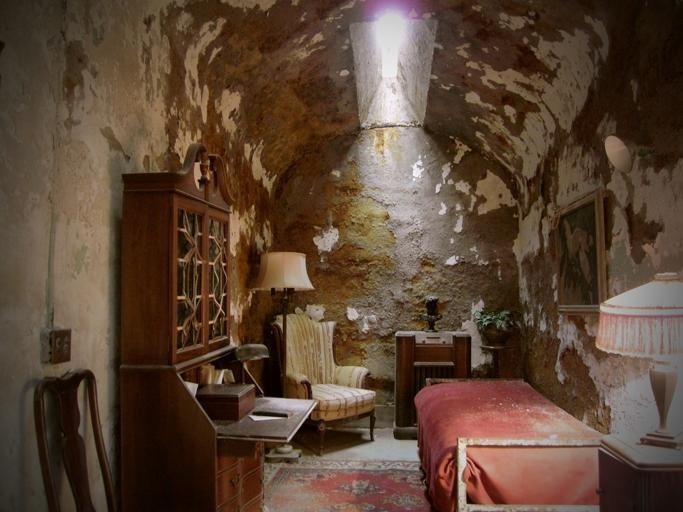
[{"left": 597, "top": 433, "right": 683, "bottom": 512}]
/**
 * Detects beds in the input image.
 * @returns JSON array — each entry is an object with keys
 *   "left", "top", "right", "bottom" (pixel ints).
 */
[{"left": 415, "top": 375, "right": 606, "bottom": 512}]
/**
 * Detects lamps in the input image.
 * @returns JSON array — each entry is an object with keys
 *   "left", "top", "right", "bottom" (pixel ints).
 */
[
  {"left": 602, "top": 135, "right": 638, "bottom": 176},
  {"left": 595, "top": 274, "right": 683, "bottom": 449},
  {"left": 251, "top": 251, "right": 315, "bottom": 340}
]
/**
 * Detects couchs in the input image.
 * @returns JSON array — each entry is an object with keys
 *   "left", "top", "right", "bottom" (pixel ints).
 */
[{"left": 270, "top": 313, "right": 377, "bottom": 454}]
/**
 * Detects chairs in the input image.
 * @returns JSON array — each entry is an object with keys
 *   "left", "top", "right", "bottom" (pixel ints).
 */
[{"left": 34, "top": 368, "right": 117, "bottom": 512}]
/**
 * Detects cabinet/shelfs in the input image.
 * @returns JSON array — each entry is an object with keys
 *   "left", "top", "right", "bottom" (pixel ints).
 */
[
  {"left": 119, "top": 345, "right": 320, "bottom": 512},
  {"left": 121, "top": 143, "right": 234, "bottom": 363}
]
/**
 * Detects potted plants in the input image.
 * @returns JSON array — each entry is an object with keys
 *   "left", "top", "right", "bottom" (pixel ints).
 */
[{"left": 474, "top": 307, "right": 518, "bottom": 345}]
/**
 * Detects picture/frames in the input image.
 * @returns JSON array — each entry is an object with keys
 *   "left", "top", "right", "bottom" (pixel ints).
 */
[{"left": 548, "top": 188, "right": 606, "bottom": 313}]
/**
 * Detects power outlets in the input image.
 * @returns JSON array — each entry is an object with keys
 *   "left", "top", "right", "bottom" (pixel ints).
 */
[{"left": 42, "top": 325, "right": 70, "bottom": 363}]
